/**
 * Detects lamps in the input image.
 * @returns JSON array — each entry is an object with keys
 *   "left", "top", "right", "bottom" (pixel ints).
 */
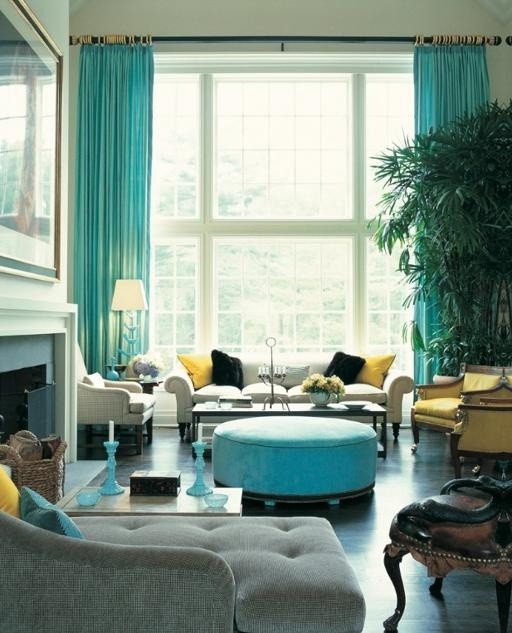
[{"left": 110, "top": 276, "right": 149, "bottom": 358}]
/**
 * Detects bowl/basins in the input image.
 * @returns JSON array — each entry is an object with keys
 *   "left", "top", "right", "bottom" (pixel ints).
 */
[
  {"left": 204, "top": 493, "right": 229, "bottom": 507},
  {"left": 76, "top": 491, "right": 100, "bottom": 506}
]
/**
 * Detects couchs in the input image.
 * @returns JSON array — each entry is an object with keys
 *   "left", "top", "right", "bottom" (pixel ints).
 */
[
  {"left": 0, "top": 514, "right": 366, "bottom": 632},
  {"left": 165, "top": 357, "right": 414, "bottom": 440}
]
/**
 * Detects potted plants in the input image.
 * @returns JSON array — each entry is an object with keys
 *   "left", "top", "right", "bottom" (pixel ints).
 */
[{"left": 363, "top": 96, "right": 512, "bottom": 385}]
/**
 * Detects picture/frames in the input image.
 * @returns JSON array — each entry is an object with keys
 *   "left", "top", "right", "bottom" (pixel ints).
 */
[{"left": 0, "top": 0, "right": 65, "bottom": 284}]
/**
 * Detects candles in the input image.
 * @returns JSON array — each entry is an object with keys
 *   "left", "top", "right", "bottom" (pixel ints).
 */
[
  {"left": 197, "top": 422, "right": 203, "bottom": 443},
  {"left": 108, "top": 419, "right": 115, "bottom": 441}
]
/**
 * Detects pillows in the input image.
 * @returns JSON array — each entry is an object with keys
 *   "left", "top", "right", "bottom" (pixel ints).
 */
[
  {"left": 210, "top": 349, "right": 245, "bottom": 389},
  {"left": 17, "top": 484, "right": 87, "bottom": 539},
  {"left": 323, "top": 351, "right": 366, "bottom": 384},
  {"left": 1, "top": 470, "right": 20, "bottom": 518},
  {"left": 354, "top": 351, "right": 396, "bottom": 389},
  {"left": 176, "top": 353, "right": 214, "bottom": 389}
]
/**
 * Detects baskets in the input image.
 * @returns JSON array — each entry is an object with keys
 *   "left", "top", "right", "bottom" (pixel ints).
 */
[{"left": 1, "top": 430, "right": 67, "bottom": 505}]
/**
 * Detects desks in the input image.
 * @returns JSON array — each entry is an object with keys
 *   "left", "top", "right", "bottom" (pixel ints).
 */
[{"left": 108, "top": 376, "right": 165, "bottom": 442}]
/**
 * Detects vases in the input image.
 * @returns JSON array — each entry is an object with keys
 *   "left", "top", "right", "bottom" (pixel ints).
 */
[
  {"left": 309, "top": 392, "right": 332, "bottom": 408},
  {"left": 141, "top": 374, "right": 153, "bottom": 382}
]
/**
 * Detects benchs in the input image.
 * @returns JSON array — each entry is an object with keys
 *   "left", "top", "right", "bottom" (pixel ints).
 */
[{"left": 210, "top": 414, "right": 379, "bottom": 508}]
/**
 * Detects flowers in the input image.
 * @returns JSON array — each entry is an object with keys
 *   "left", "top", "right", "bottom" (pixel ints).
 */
[
  {"left": 297, "top": 372, "right": 345, "bottom": 402},
  {"left": 135, "top": 354, "right": 162, "bottom": 378}
]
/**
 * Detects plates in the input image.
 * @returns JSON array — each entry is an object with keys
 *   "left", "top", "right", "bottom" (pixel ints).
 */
[{"left": 344, "top": 403, "right": 365, "bottom": 408}]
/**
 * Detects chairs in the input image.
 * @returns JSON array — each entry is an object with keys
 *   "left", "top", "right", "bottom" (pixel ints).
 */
[
  {"left": 77, "top": 373, "right": 156, "bottom": 456},
  {"left": 410, "top": 360, "right": 512, "bottom": 452},
  {"left": 381, "top": 471, "right": 512, "bottom": 633},
  {"left": 449, "top": 380, "right": 512, "bottom": 485}
]
